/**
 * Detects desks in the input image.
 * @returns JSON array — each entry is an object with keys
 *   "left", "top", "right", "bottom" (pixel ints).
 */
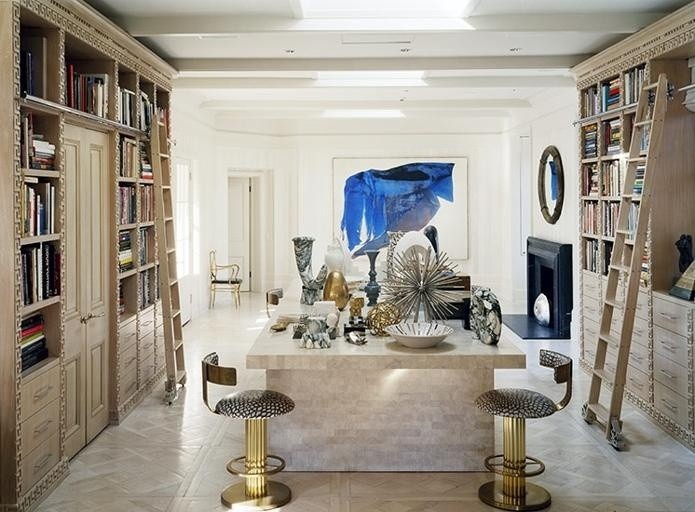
[{"left": 245, "top": 290, "right": 526, "bottom": 473}]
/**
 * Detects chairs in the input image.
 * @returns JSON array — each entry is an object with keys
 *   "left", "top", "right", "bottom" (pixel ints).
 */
[
  {"left": 208, "top": 250, "right": 243, "bottom": 309},
  {"left": 266, "top": 288, "right": 284, "bottom": 318},
  {"left": 473, "top": 348, "right": 572, "bottom": 510},
  {"left": 201, "top": 351, "right": 295, "bottom": 512}
]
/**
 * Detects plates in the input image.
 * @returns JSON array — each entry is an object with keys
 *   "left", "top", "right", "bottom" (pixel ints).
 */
[{"left": 384, "top": 322, "right": 454, "bottom": 348}]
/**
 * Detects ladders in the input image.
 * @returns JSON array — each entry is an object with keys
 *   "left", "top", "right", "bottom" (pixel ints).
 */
[
  {"left": 147, "top": 114, "right": 187, "bottom": 401},
  {"left": 581, "top": 73, "right": 673, "bottom": 451}
]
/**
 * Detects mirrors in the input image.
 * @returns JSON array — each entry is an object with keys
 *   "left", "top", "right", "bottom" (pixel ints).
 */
[{"left": 537, "top": 145, "right": 563, "bottom": 225}]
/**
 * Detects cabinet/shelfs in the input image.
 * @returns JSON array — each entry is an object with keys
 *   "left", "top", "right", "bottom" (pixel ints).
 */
[
  {"left": 0, "top": 1, "right": 178, "bottom": 512},
  {"left": 570, "top": 1, "right": 695, "bottom": 458}
]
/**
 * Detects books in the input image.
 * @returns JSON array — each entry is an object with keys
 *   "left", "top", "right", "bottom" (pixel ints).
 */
[
  {"left": 19, "top": 52, "right": 33, "bottom": 98},
  {"left": 66, "top": 65, "right": 109, "bottom": 119},
  {"left": 19, "top": 176, "right": 55, "bottom": 237},
  {"left": 120, "top": 137, "right": 153, "bottom": 179},
  {"left": 119, "top": 228, "right": 150, "bottom": 273},
  {"left": 20, "top": 111, "right": 56, "bottom": 171},
  {"left": 20, "top": 241, "right": 60, "bottom": 307},
  {"left": 118, "top": 184, "right": 154, "bottom": 224},
  {"left": 19, "top": 314, "right": 48, "bottom": 372},
  {"left": 140, "top": 271, "right": 150, "bottom": 309},
  {"left": 118, "top": 88, "right": 154, "bottom": 132},
  {"left": 579, "top": 62, "right": 656, "bottom": 286}
]
